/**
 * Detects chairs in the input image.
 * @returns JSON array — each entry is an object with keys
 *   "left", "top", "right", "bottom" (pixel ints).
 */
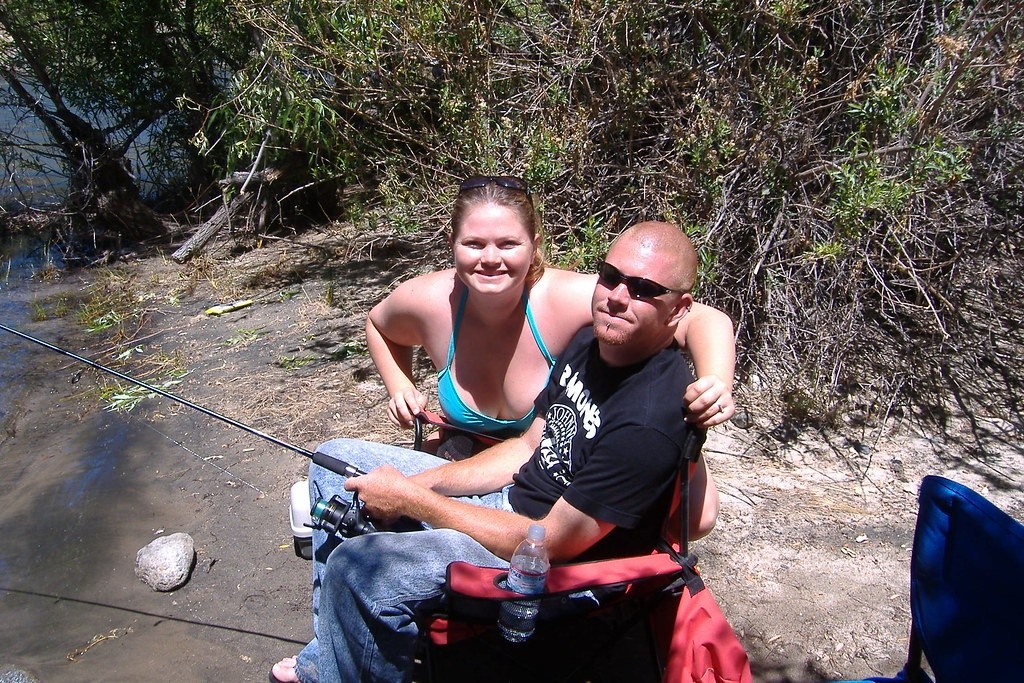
[
  {"left": 833, "top": 474, "right": 1024, "bottom": 683},
  {"left": 409, "top": 406, "right": 750, "bottom": 683}
]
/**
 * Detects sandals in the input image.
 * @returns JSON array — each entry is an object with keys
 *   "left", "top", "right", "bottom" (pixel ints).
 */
[{"left": 269, "top": 655, "right": 303, "bottom": 683}]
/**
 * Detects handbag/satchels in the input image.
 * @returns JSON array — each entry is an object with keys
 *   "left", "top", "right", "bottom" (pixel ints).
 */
[{"left": 657, "top": 535, "right": 754, "bottom": 683}]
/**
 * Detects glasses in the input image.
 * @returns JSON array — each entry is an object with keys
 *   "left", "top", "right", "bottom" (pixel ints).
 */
[
  {"left": 458, "top": 176, "right": 532, "bottom": 201},
  {"left": 596, "top": 261, "right": 685, "bottom": 299}
]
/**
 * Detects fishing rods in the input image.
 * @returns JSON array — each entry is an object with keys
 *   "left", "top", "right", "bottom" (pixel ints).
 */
[{"left": 1, "top": 324, "right": 375, "bottom": 542}]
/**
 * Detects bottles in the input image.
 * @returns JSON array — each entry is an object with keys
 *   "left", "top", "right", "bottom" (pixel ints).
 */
[{"left": 495, "top": 524, "right": 550, "bottom": 643}]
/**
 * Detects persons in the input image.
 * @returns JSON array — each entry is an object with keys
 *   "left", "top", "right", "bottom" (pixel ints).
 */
[
  {"left": 272, "top": 221, "right": 709, "bottom": 683},
  {"left": 366, "top": 176, "right": 735, "bottom": 463}
]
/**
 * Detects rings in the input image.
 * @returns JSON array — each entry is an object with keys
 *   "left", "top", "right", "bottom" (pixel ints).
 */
[{"left": 718, "top": 405, "right": 725, "bottom": 413}]
